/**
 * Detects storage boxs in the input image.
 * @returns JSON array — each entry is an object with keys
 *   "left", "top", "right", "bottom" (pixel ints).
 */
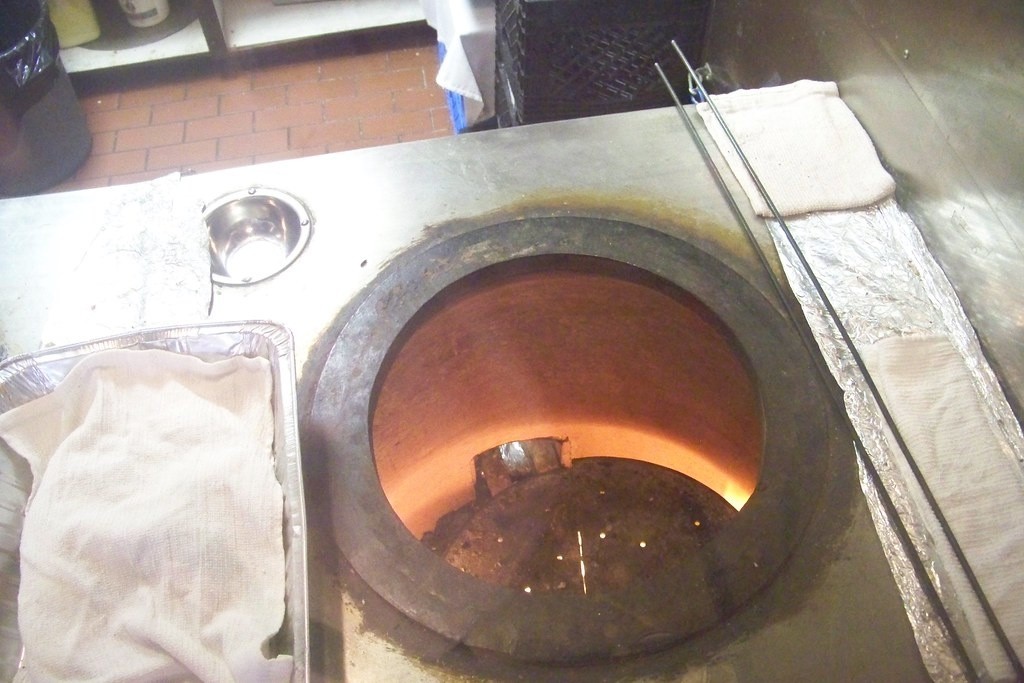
[{"left": 437, "top": 0, "right": 710, "bottom": 136}]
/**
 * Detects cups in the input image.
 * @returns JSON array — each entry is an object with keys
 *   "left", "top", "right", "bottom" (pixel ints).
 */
[{"left": 118, "top": 0, "right": 170, "bottom": 27}]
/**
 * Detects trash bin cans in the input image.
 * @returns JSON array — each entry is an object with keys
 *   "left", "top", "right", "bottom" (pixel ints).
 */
[{"left": 0, "top": 0, "right": 93, "bottom": 199}]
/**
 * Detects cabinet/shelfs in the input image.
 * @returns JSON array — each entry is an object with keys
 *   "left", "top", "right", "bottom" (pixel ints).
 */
[{"left": 47, "top": 1, "right": 444, "bottom": 100}]
